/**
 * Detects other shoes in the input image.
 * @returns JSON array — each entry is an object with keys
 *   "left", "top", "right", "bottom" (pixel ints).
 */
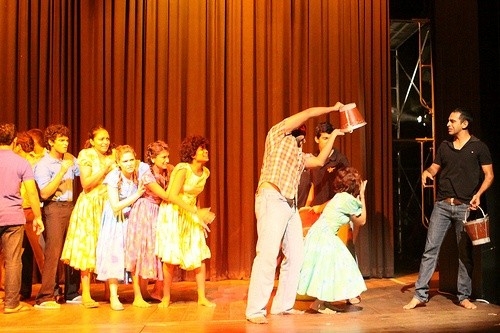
[
  {"left": 111, "top": 295, "right": 124, "bottom": 311},
  {"left": 133, "top": 297, "right": 150, "bottom": 307}
]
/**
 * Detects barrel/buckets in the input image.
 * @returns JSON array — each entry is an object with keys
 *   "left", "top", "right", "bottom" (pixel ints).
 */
[
  {"left": 339, "top": 103, "right": 367, "bottom": 133},
  {"left": 462, "top": 204, "right": 491, "bottom": 246}
]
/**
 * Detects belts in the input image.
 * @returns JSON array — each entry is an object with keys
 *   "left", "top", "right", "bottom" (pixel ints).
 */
[
  {"left": 444, "top": 198, "right": 463, "bottom": 204},
  {"left": 269, "top": 182, "right": 295, "bottom": 205}
]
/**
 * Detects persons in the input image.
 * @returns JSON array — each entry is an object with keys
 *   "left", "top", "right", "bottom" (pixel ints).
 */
[
  {"left": 245, "top": 102, "right": 345, "bottom": 324},
  {"left": 0, "top": 122, "right": 216, "bottom": 313},
  {"left": 297, "top": 123, "right": 348, "bottom": 214},
  {"left": 403, "top": 108, "right": 495, "bottom": 310},
  {"left": 296, "top": 167, "right": 369, "bottom": 314}
]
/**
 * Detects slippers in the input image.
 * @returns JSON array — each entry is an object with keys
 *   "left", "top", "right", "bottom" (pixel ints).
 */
[
  {"left": 34, "top": 301, "right": 60, "bottom": 308},
  {"left": 80, "top": 299, "right": 99, "bottom": 307},
  {"left": 66, "top": 296, "right": 81, "bottom": 304},
  {"left": 5, "top": 305, "right": 31, "bottom": 314}
]
